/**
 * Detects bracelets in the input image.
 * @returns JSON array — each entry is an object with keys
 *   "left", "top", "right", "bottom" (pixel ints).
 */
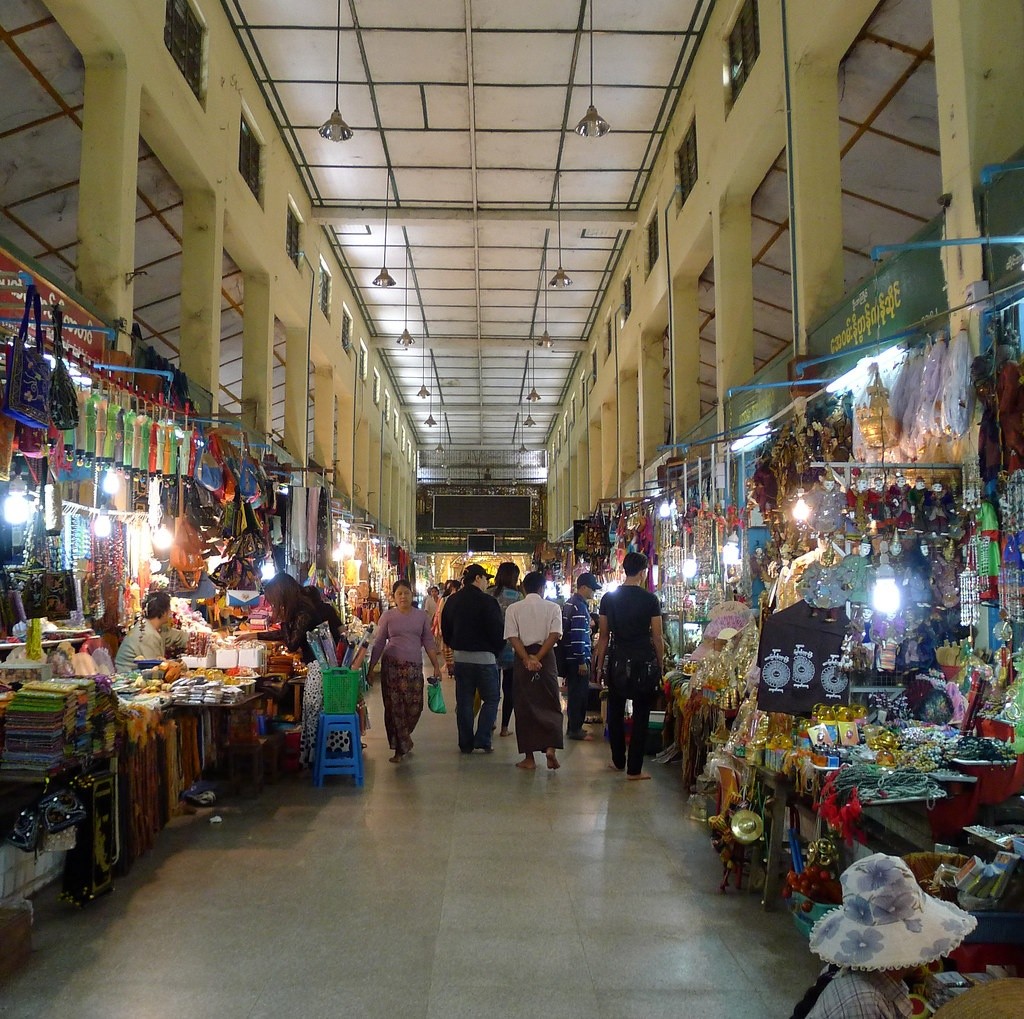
[{"left": 596, "top": 667, "right": 604, "bottom": 673}]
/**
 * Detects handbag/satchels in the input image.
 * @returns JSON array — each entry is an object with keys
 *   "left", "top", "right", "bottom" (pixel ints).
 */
[
  {"left": 6, "top": 512, "right": 77, "bottom": 620},
  {"left": 0, "top": 381, "right": 17, "bottom": 481},
  {"left": 166, "top": 425, "right": 276, "bottom": 606},
  {"left": 554, "top": 646, "right": 567, "bottom": 677},
  {"left": 611, "top": 646, "right": 662, "bottom": 694},
  {"left": 50, "top": 304, "right": 79, "bottom": 430},
  {"left": 532, "top": 542, "right": 570, "bottom": 566},
  {"left": 2, "top": 285, "right": 51, "bottom": 431},
  {"left": 427, "top": 681, "right": 446, "bottom": 715},
  {"left": 573, "top": 500, "right": 647, "bottom": 572},
  {"left": 6, "top": 345, "right": 47, "bottom": 458}
]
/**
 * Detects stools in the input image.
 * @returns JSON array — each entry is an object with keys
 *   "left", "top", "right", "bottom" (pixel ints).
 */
[{"left": 313, "top": 713, "right": 366, "bottom": 787}]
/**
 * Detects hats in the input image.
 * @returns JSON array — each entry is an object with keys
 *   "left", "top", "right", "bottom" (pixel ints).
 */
[
  {"left": 463, "top": 564, "right": 494, "bottom": 579},
  {"left": 576, "top": 574, "right": 603, "bottom": 589},
  {"left": 810, "top": 853, "right": 979, "bottom": 971}
]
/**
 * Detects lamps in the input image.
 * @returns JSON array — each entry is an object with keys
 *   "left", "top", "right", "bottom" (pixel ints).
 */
[
  {"left": 510, "top": 437, "right": 519, "bottom": 486},
  {"left": 318, "top": 0, "right": 354, "bottom": 141},
  {"left": 548, "top": 170, "right": 573, "bottom": 288},
  {"left": 518, "top": 397, "right": 528, "bottom": 455},
  {"left": 424, "top": 358, "right": 438, "bottom": 427},
  {"left": 417, "top": 321, "right": 431, "bottom": 400},
  {"left": 526, "top": 322, "right": 542, "bottom": 402},
  {"left": 446, "top": 438, "right": 453, "bottom": 487},
  {"left": 393, "top": 245, "right": 415, "bottom": 348},
  {"left": 440, "top": 416, "right": 449, "bottom": 470},
  {"left": 524, "top": 357, "right": 536, "bottom": 427},
  {"left": 537, "top": 248, "right": 554, "bottom": 348},
  {"left": 435, "top": 394, "right": 445, "bottom": 454},
  {"left": 373, "top": 170, "right": 397, "bottom": 288},
  {"left": 573, "top": 0, "right": 611, "bottom": 139}
]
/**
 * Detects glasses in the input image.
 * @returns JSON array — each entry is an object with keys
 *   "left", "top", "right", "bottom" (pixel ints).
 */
[{"left": 587, "top": 586, "right": 595, "bottom": 591}]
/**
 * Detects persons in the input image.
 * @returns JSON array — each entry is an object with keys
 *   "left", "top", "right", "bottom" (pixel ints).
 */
[
  {"left": 115, "top": 592, "right": 190, "bottom": 674},
  {"left": 441, "top": 564, "right": 505, "bottom": 754},
  {"left": 504, "top": 571, "right": 567, "bottom": 770},
  {"left": 366, "top": 579, "right": 443, "bottom": 762},
  {"left": 561, "top": 573, "right": 603, "bottom": 741},
  {"left": 803, "top": 852, "right": 978, "bottom": 1019},
  {"left": 595, "top": 551, "right": 665, "bottom": 781},
  {"left": 424, "top": 569, "right": 506, "bottom": 683},
  {"left": 303, "top": 585, "right": 371, "bottom": 750},
  {"left": 486, "top": 562, "right": 528, "bottom": 737},
  {"left": 233, "top": 573, "right": 341, "bottom": 767}
]
